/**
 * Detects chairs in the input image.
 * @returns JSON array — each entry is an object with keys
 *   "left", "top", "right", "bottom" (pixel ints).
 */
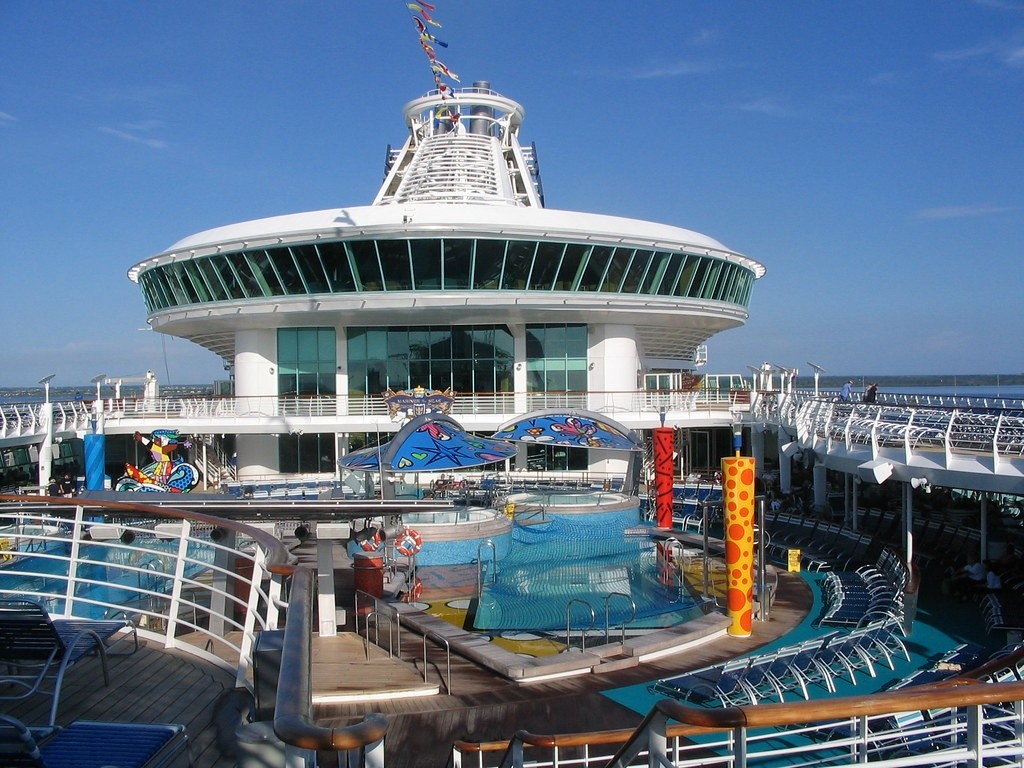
[
  {"left": 638, "top": 483, "right": 1024, "bottom": 768},
  {"left": 0, "top": 598, "right": 197, "bottom": 768},
  {"left": 832, "top": 402, "right": 1024, "bottom": 456},
  {"left": 428, "top": 476, "right": 624, "bottom": 499}
]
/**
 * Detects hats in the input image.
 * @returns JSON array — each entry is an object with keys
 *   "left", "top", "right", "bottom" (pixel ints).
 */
[{"left": 51, "top": 479, "right": 56, "bottom": 482}]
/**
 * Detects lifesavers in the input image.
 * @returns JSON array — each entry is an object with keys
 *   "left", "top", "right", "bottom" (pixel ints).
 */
[
  {"left": 360, "top": 533, "right": 380, "bottom": 552},
  {"left": 403, "top": 576, "right": 423, "bottom": 603},
  {"left": 395, "top": 529, "right": 422, "bottom": 556}
]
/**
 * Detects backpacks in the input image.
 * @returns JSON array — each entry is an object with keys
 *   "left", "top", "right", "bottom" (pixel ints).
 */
[{"left": 63, "top": 485, "right": 71, "bottom": 494}]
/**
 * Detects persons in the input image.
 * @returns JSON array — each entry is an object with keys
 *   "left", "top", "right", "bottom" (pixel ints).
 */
[
  {"left": 0, "top": 459, "right": 81, "bottom": 499},
  {"left": 760, "top": 468, "right": 1024, "bottom": 610},
  {"left": 431, "top": 475, "right": 448, "bottom": 501},
  {"left": 837, "top": 380, "right": 878, "bottom": 404},
  {"left": 685, "top": 471, "right": 722, "bottom": 488}
]
